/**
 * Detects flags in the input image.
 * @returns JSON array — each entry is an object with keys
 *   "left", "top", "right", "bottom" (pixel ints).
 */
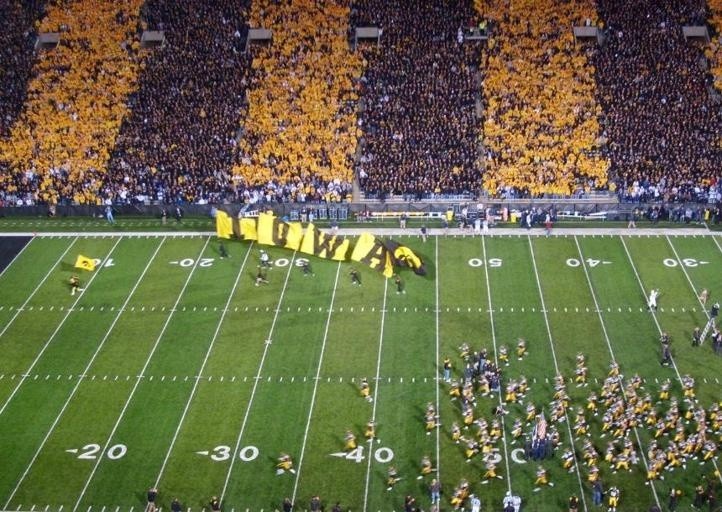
[{"left": 76, "top": 255, "right": 96, "bottom": 271}]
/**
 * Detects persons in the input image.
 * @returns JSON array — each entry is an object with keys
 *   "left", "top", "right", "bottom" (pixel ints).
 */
[
  {"left": 345, "top": 379, "right": 400, "bottom": 490},
  {"left": 405, "top": 338, "right": 722, "bottom": 512},
  {"left": 276, "top": 451, "right": 296, "bottom": 473},
  {"left": 148, "top": 488, "right": 221, "bottom": 512},
  {"left": 352, "top": 268, "right": 362, "bottom": 286},
  {"left": 255, "top": 249, "right": 272, "bottom": 286},
  {"left": 70, "top": 274, "right": 85, "bottom": 295},
  {"left": 283, "top": 495, "right": 341, "bottom": 512},
  {"left": 393, "top": 272, "right": 402, "bottom": 292},
  {"left": 303, "top": 261, "right": 315, "bottom": 277},
  {"left": 0, "top": 1, "right": 722, "bottom": 242},
  {"left": 219, "top": 241, "right": 229, "bottom": 259},
  {"left": 648, "top": 286, "right": 722, "bottom": 362}
]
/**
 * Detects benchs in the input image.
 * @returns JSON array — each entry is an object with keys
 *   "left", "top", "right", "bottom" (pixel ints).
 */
[
  {"left": 354, "top": 212, "right": 447, "bottom": 223},
  {"left": 556, "top": 211, "right": 607, "bottom": 222}
]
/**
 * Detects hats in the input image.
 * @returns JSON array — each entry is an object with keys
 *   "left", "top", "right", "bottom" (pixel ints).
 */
[{"left": 260, "top": 249, "right": 264, "bottom": 253}]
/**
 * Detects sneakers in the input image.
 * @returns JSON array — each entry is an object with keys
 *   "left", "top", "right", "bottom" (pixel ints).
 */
[
  {"left": 386, "top": 478, "right": 400, "bottom": 490},
  {"left": 416, "top": 352, "right": 722, "bottom": 511},
  {"left": 290, "top": 469, "right": 295, "bottom": 475}
]
